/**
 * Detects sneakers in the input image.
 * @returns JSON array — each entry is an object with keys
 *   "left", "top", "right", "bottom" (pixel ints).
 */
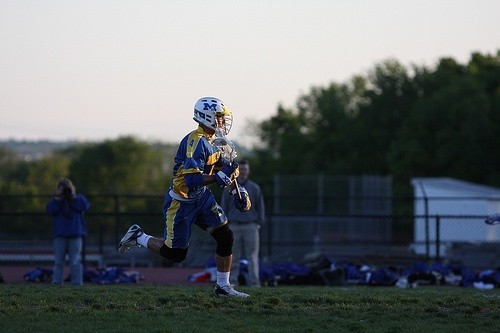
[
  {"left": 119, "top": 224, "right": 144, "bottom": 254},
  {"left": 215, "top": 283, "right": 250, "bottom": 298}
]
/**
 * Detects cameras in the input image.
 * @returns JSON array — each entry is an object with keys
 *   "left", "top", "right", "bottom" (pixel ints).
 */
[{"left": 63, "top": 185, "right": 71, "bottom": 196}]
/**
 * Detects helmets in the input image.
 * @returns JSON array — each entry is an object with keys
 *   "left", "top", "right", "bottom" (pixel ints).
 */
[{"left": 193, "top": 97, "right": 232, "bottom": 135}]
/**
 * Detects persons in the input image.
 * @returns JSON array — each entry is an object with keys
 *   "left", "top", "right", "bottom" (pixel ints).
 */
[
  {"left": 118, "top": 97, "right": 251, "bottom": 300},
  {"left": 220, "top": 161, "right": 265, "bottom": 289},
  {"left": 48, "top": 179, "right": 91, "bottom": 286}
]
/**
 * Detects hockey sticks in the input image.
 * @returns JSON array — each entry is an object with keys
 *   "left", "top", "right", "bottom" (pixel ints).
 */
[
  {"left": 484, "top": 214, "right": 500, "bottom": 225},
  {"left": 211, "top": 137, "right": 242, "bottom": 200}
]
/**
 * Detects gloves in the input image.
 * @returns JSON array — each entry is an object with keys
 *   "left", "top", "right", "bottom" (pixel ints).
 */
[
  {"left": 231, "top": 184, "right": 251, "bottom": 212},
  {"left": 216, "top": 160, "right": 240, "bottom": 188}
]
